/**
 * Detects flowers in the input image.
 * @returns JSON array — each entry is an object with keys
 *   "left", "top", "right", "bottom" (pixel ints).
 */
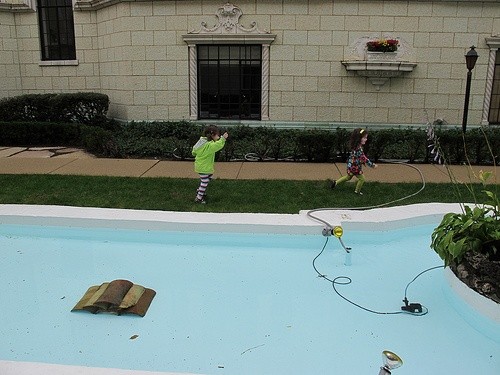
[{"left": 366, "top": 39, "right": 398, "bottom": 52}]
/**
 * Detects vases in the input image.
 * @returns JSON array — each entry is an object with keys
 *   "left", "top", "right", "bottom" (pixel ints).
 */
[{"left": 366, "top": 52, "right": 398, "bottom": 61}]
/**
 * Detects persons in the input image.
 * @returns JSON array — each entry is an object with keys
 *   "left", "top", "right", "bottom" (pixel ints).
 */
[
  {"left": 331, "top": 128, "right": 377, "bottom": 195},
  {"left": 192, "top": 125, "right": 228, "bottom": 205}
]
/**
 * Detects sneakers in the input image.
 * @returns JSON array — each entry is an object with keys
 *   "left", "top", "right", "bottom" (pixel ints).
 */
[
  {"left": 194, "top": 197, "right": 206, "bottom": 205},
  {"left": 331, "top": 181, "right": 336, "bottom": 190},
  {"left": 353, "top": 191, "right": 363, "bottom": 195},
  {"left": 196, "top": 192, "right": 206, "bottom": 197}
]
